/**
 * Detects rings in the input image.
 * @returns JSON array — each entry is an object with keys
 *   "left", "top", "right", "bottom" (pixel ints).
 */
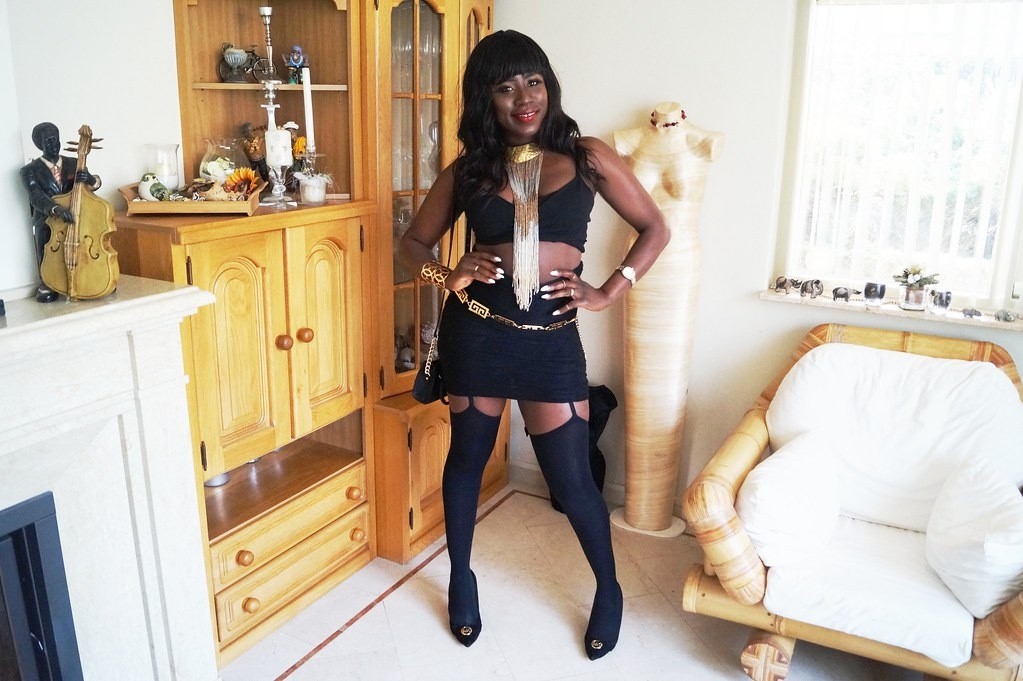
[
  {"left": 570, "top": 288, "right": 575, "bottom": 296},
  {"left": 475, "top": 266, "right": 480, "bottom": 271},
  {"left": 562, "top": 280, "right": 565, "bottom": 289},
  {"left": 565, "top": 304, "right": 570, "bottom": 310},
  {"left": 569, "top": 273, "right": 576, "bottom": 280}
]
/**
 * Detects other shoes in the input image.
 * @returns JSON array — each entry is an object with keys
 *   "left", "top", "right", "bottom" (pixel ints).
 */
[
  {"left": 585, "top": 582, "right": 623, "bottom": 660},
  {"left": 448, "top": 569, "right": 482, "bottom": 647}
]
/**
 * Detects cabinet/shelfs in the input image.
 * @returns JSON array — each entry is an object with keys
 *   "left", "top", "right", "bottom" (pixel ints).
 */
[
  {"left": 0, "top": 273, "right": 218, "bottom": 681},
  {"left": 110, "top": 0, "right": 510, "bottom": 667}
]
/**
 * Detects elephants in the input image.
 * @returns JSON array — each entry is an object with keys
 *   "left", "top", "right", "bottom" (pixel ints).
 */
[
  {"left": 774, "top": 276, "right": 824, "bottom": 299},
  {"left": 832, "top": 282, "right": 863, "bottom": 302},
  {"left": 962, "top": 308, "right": 982, "bottom": 319}
]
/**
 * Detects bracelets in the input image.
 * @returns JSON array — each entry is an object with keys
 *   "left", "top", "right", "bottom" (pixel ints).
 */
[{"left": 420, "top": 260, "right": 453, "bottom": 289}]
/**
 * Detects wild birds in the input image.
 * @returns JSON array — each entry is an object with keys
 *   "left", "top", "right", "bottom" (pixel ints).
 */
[{"left": 137, "top": 173, "right": 167, "bottom": 202}]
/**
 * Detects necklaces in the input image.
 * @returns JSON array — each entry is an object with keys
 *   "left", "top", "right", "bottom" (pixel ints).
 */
[
  {"left": 502, "top": 141, "right": 545, "bottom": 312},
  {"left": 649, "top": 110, "right": 687, "bottom": 129}
]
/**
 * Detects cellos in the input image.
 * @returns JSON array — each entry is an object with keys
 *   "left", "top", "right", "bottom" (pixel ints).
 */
[{"left": 39, "top": 124, "right": 119, "bottom": 298}]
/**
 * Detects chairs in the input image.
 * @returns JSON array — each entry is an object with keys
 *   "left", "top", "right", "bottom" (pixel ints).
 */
[{"left": 681, "top": 323, "right": 1023, "bottom": 681}]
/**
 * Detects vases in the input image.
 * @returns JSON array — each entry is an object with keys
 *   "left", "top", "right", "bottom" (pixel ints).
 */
[{"left": 898, "top": 287, "right": 928, "bottom": 311}]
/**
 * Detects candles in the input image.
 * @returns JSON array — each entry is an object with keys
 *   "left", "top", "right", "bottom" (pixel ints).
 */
[{"left": 302, "top": 68, "right": 315, "bottom": 152}]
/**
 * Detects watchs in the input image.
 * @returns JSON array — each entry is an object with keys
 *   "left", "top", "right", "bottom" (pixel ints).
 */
[{"left": 615, "top": 265, "right": 637, "bottom": 288}]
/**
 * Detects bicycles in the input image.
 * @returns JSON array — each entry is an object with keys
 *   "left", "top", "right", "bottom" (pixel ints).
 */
[{"left": 216, "top": 43, "right": 278, "bottom": 84}]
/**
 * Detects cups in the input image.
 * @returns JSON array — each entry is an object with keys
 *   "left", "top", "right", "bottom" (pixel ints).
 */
[
  {"left": 928, "top": 289, "right": 951, "bottom": 315},
  {"left": 864, "top": 282, "right": 886, "bottom": 312},
  {"left": 300, "top": 181, "right": 328, "bottom": 206}
]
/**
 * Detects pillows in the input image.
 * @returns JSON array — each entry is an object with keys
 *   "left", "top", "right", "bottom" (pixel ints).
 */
[
  {"left": 923, "top": 433, "right": 1023, "bottom": 621},
  {"left": 734, "top": 424, "right": 834, "bottom": 567}
]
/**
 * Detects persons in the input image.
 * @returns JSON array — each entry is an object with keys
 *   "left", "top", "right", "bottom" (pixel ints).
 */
[
  {"left": 282, "top": 120, "right": 307, "bottom": 194},
  {"left": 398, "top": 30, "right": 672, "bottom": 661},
  {"left": 613, "top": 102, "right": 725, "bottom": 532},
  {"left": 20, "top": 122, "right": 117, "bottom": 303},
  {"left": 280, "top": 45, "right": 310, "bottom": 84},
  {"left": 241, "top": 122, "right": 272, "bottom": 192}
]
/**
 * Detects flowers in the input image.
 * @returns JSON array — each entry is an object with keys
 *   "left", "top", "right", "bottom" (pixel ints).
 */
[
  {"left": 891, "top": 263, "right": 943, "bottom": 287},
  {"left": 223, "top": 166, "right": 259, "bottom": 193}
]
